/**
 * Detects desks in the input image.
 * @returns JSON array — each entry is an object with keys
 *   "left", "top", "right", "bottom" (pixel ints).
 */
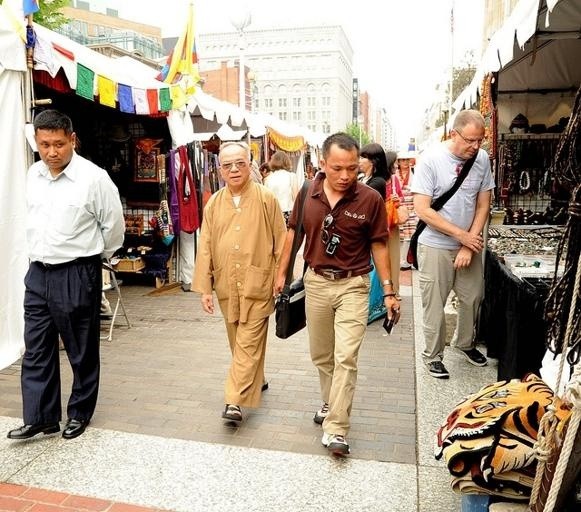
[{"left": 477, "top": 225, "right": 571, "bottom": 382}]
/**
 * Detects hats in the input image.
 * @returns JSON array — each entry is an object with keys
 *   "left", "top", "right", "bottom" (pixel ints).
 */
[
  {"left": 110, "top": 127, "right": 132, "bottom": 141},
  {"left": 397, "top": 152, "right": 417, "bottom": 159}
]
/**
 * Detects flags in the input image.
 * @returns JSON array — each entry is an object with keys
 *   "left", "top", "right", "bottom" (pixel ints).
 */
[
  {"left": 75, "top": 62, "right": 95, "bottom": 101},
  {"left": 98, "top": 75, "right": 116, "bottom": 109},
  {"left": 117, "top": 83, "right": 134, "bottom": 114},
  {"left": 133, "top": 83, "right": 190, "bottom": 116}
]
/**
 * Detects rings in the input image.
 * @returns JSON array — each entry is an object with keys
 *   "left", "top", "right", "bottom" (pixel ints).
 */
[{"left": 478, "top": 240, "right": 481, "bottom": 245}]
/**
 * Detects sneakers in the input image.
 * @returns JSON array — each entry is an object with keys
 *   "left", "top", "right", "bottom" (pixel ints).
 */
[
  {"left": 322, "top": 433, "right": 350, "bottom": 454},
  {"left": 423, "top": 362, "right": 450, "bottom": 377},
  {"left": 315, "top": 403, "right": 328, "bottom": 423},
  {"left": 459, "top": 348, "right": 488, "bottom": 366}
]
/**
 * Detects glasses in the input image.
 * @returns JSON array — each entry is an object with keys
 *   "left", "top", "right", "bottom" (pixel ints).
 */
[
  {"left": 456, "top": 130, "right": 483, "bottom": 144},
  {"left": 220, "top": 160, "right": 246, "bottom": 169},
  {"left": 321, "top": 213, "right": 333, "bottom": 244}
]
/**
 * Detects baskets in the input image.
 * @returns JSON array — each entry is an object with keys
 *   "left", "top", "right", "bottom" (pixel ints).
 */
[{"left": 112, "top": 257, "right": 145, "bottom": 272}]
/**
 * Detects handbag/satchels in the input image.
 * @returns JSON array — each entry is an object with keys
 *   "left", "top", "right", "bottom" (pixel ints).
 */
[
  {"left": 386, "top": 199, "right": 398, "bottom": 225},
  {"left": 397, "top": 206, "right": 409, "bottom": 224},
  {"left": 275, "top": 277, "right": 306, "bottom": 339},
  {"left": 156, "top": 188, "right": 212, "bottom": 246},
  {"left": 407, "top": 234, "right": 418, "bottom": 271}
]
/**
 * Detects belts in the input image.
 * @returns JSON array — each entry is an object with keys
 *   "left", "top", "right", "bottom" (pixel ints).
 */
[
  {"left": 35, "top": 258, "right": 85, "bottom": 269},
  {"left": 308, "top": 265, "right": 374, "bottom": 280}
]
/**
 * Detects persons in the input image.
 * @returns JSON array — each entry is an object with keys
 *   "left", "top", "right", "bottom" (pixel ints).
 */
[
  {"left": 5, "top": 108, "right": 126, "bottom": 441},
  {"left": 190, "top": 141, "right": 288, "bottom": 422},
  {"left": 409, "top": 109, "right": 498, "bottom": 379},
  {"left": 249, "top": 143, "right": 419, "bottom": 327},
  {"left": 272, "top": 132, "right": 400, "bottom": 456}
]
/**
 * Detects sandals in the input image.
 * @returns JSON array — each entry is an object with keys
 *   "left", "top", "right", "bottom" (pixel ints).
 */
[
  {"left": 261, "top": 382, "right": 267, "bottom": 390},
  {"left": 222, "top": 405, "right": 242, "bottom": 420}
]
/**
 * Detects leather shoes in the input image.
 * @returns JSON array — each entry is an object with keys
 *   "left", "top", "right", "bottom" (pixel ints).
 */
[
  {"left": 7, "top": 424, "right": 59, "bottom": 439},
  {"left": 63, "top": 419, "right": 90, "bottom": 438}
]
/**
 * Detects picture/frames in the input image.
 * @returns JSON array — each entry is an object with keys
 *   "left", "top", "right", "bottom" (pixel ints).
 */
[{"left": 133, "top": 147, "right": 160, "bottom": 182}]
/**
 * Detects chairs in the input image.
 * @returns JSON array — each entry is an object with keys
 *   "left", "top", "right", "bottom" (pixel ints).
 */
[{"left": 100, "top": 258, "right": 131, "bottom": 342}]
